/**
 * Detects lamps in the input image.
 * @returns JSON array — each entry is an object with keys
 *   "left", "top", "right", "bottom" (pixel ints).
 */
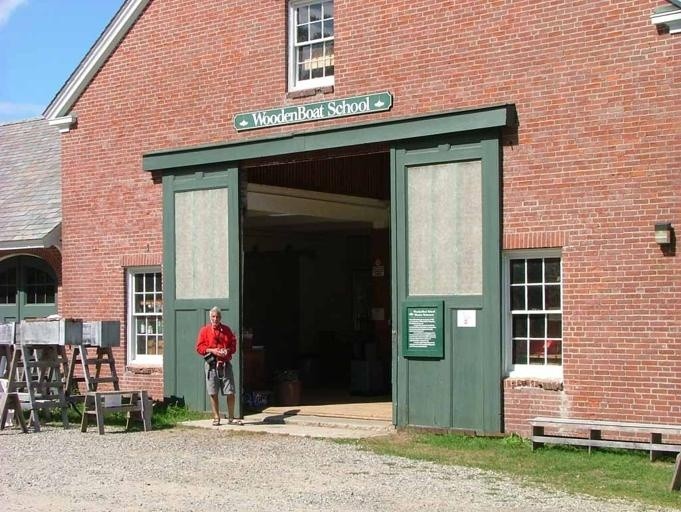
[{"left": 654, "top": 222, "right": 672, "bottom": 245}]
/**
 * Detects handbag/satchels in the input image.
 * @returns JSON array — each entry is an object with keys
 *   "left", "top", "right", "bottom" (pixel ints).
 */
[{"left": 204, "top": 352, "right": 217, "bottom": 369}]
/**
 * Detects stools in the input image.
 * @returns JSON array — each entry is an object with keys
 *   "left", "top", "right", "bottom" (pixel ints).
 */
[{"left": 80, "top": 389, "right": 155, "bottom": 435}]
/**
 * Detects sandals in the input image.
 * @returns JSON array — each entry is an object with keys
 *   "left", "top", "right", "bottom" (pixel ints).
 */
[
  {"left": 212, "top": 417, "right": 221, "bottom": 425},
  {"left": 228, "top": 420, "right": 244, "bottom": 425}
]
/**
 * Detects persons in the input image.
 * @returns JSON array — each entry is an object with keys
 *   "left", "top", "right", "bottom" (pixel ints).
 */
[{"left": 196, "top": 305, "right": 244, "bottom": 426}]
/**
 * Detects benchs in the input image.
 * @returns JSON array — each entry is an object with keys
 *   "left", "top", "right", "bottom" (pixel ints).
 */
[{"left": 526, "top": 415, "right": 681, "bottom": 463}]
